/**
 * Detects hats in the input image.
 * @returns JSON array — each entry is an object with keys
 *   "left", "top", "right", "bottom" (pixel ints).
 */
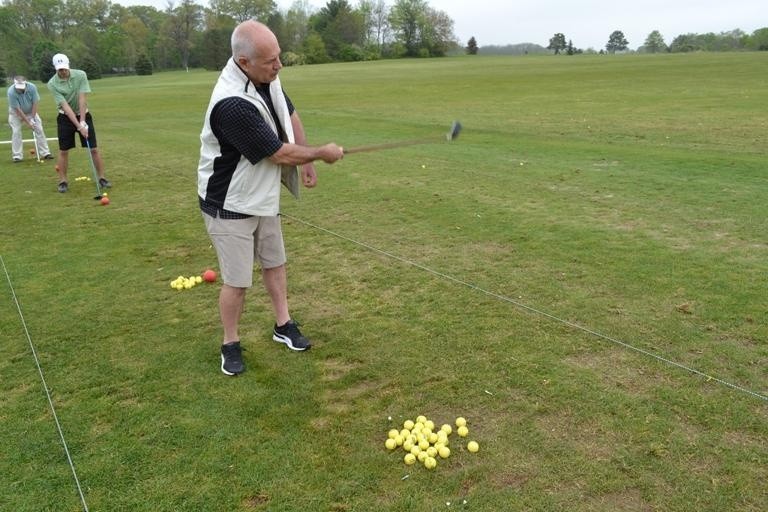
[
  {"left": 13, "top": 76, "right": 27, "bottom": 90},
  {"left": 53, "top": 53, "right": 70, "bottom": 71}
]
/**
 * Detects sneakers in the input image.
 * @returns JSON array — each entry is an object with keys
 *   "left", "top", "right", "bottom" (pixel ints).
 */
[
  {"left": 57, "top": 181, "right": 68, "bottom": 193},
  {"left": 270, "top": 318, "right": 312, "bottom": 353},
  {"left": 10, "top": 152, "right": 55, "bottom": 162},
  {"left": 98, "top": 178, "right": 113, "bottom": 188},
  {"left": 219, "top": 339, "right": 247, "bottom": 377}
]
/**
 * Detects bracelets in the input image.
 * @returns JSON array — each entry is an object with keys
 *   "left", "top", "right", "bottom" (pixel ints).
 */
[{"left": 76, "top": 126, "right": 83, "bottom": 131}]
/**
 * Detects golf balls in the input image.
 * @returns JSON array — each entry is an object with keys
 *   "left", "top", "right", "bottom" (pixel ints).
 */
[
  {"left": 40, "top": 160, "right": 44, "bottom": 163},
  {"left": 385, "top": 415, "right": 479, "bottom": 469},
  {"left": 30, "top": 148, "right": 34, "bottom": 153},
  {"left": 102, "top": 197, "right": 110, "bottom": 205},
  {"left": 75, "top": 176, "right": 91, "bottom": 182},
  {"left": 170, "top": 270, "right": 216, "bottom": 290},
  {"left": 103, "top": 192, "right": 107, "bottom": 196}
]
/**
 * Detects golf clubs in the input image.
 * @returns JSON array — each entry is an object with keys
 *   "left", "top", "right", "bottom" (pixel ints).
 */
[
  {"left": 83, "top": 137, "right": 103, "bottom": 200},
  {"left": 33, "top": 128, "right": 41, "bottom": 162},
  {"left": 344, "top": 122, "right": 462, "bottom": 154}
]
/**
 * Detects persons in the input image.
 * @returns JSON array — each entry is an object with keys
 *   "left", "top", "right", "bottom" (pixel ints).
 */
[
  {"left": 48, "top": 54, "right": 112, "bottom": 192},
  {"left": 198, "top": 19, "right": 343, "bottom": 376},
  {"left": 7, "top": 74, "right": 54, "bottom": 162}
]
